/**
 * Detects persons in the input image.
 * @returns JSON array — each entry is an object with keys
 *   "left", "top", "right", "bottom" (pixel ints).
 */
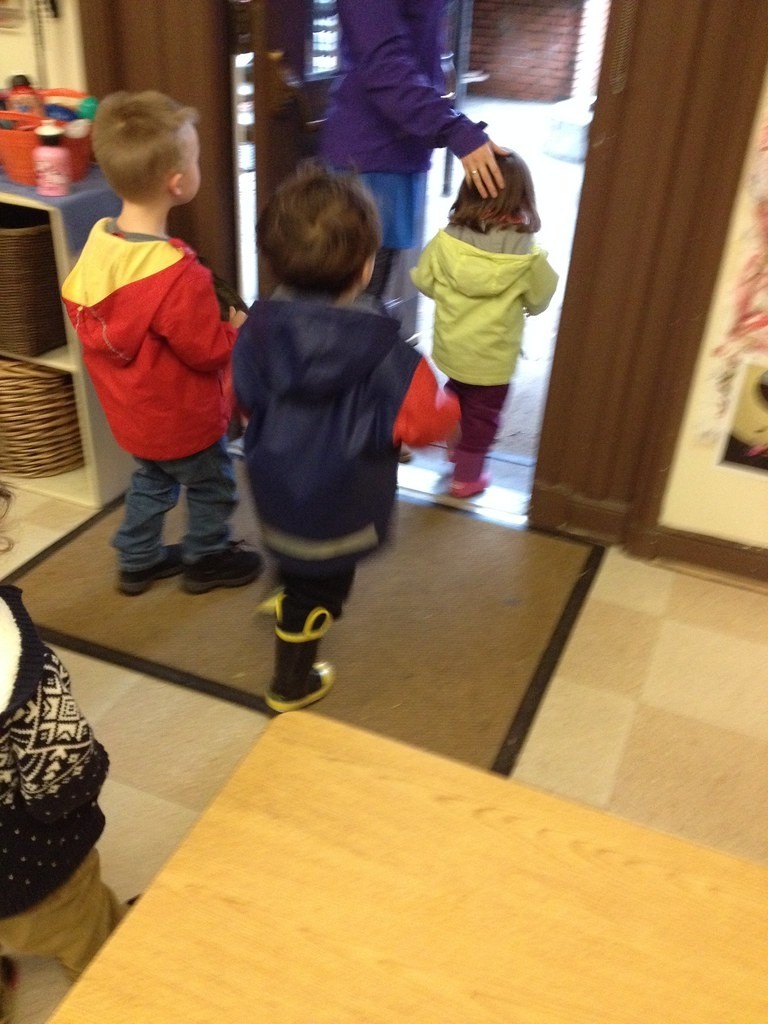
[
  {"left": 61, "top": 89, "right": 265, "bottom": 595},
  {"left": 225, "top": 156, "right": 460, "bottom": 713},
  {"left": 318, "top": 0, "right": 513, "bottom": 464},
  {"left": 410, "top": 148, "right": 559, "bottom": 500},
  {"left": 0, "top": 482, "right": 141, "bottom": 1024}
]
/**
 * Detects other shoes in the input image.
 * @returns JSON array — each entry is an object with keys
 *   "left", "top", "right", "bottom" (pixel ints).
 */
[
  {"left": 450, "top": 472, "right": 490, "bottom": 497},
  {"left": 184, "top": 540, "right": 264, "bottom": 593},
  {"left": 448, "top": 449, "right": 456, "bottom": 462},
  {"left": 116, "top": 543, "right": 183, "bottom": 593},
  {"left": 396, "top": 445, "right": 414, "bottom": 461}
]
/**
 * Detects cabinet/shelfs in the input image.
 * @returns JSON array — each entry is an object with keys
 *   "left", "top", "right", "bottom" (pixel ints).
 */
[{"left": 0, "top": 162, "right": 143, "bottom": 511}]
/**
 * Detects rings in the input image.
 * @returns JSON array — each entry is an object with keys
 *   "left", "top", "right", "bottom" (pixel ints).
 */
[{"left": 471, "top": 170, "right": 478, "bottom": 174}]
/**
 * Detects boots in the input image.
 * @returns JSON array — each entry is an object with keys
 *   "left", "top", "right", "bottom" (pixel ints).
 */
[{"left": 265, "top": 596, "right": 334, "bottom": 712}]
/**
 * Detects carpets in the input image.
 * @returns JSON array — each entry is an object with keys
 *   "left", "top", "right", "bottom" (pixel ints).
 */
[{"left": 0, "top": 452, "right": 608, "bottom": 778}]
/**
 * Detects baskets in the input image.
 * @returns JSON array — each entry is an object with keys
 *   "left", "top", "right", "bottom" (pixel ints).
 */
[
  {"left": 0, "top": 87, "right": 96, "bottom": 185},
  {"left": 0, "top": 224, "right": 63, "bottom": 357},
  {"left": 0, "top": 361, "right": 83, "bottom": 481}
]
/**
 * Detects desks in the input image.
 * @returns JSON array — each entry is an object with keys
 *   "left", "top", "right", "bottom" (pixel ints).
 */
[{"left": 45, "top": 709, "right": 768, "bottom": 1024}]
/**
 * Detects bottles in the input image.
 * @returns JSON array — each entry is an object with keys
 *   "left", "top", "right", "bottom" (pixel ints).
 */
[
  {"left": 32, "top": 119, "right": 72, "bottom": 197},
  {"left": 5, "top": 75, "right": 46, "bottom": 116}
]
